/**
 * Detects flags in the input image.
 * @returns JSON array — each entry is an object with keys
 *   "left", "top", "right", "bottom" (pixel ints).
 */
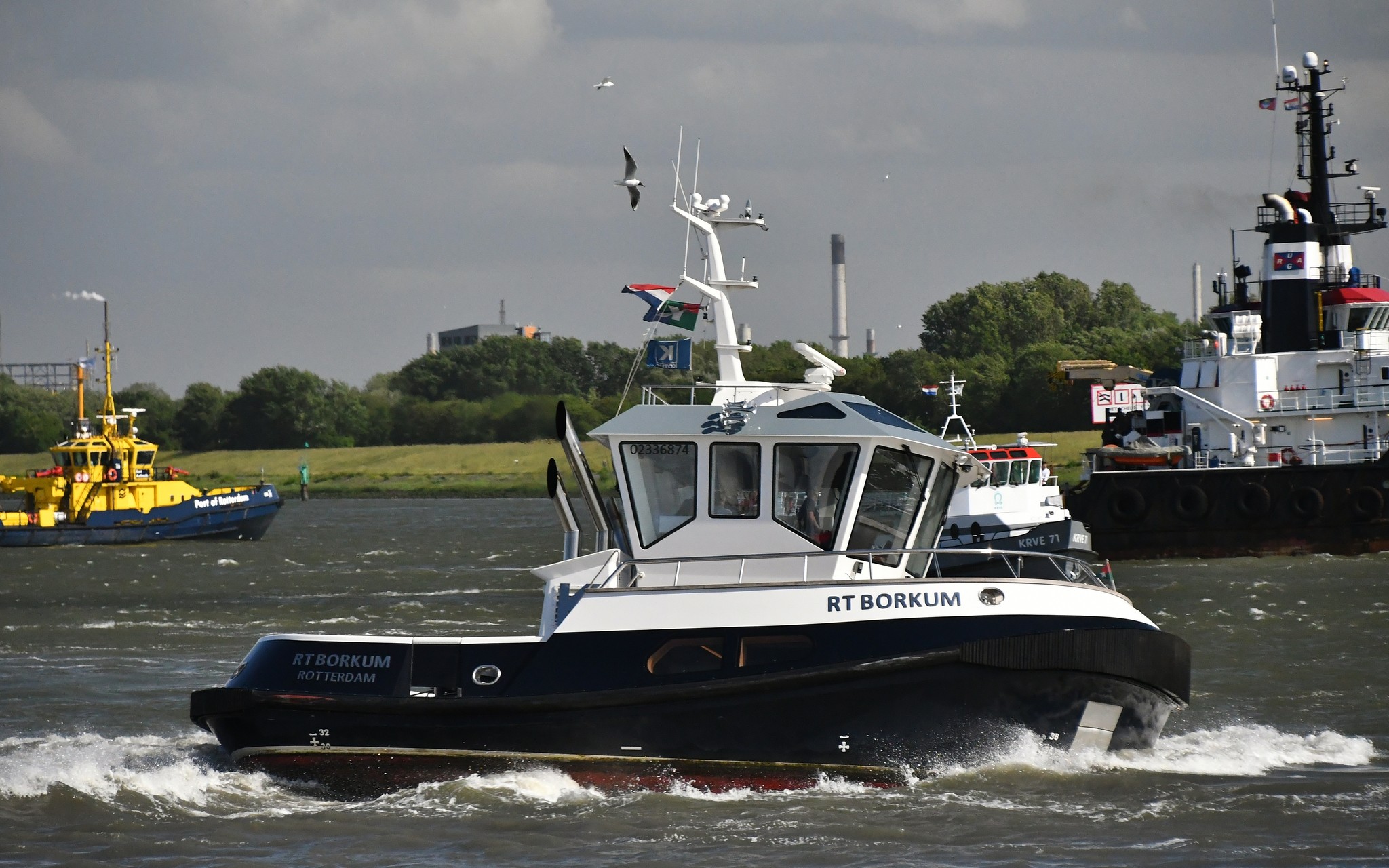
[
  {"left": 645, "top": 336, "right": 692, "bottom": 371},
  {"left": 1284, "top": 97, "right": 1301, "bottom": 110},
  {"left": 622, "top": 282, "right": 678, "bottom": 311},
  {"left": 1259, "top": 97, "right": 1277, "bottom": 110},
  {"left": 643, "top": 298, "right": 699, "bottom": 330},
  {"left": 921, "top": 384, "right": 939, "bottom": 396}
]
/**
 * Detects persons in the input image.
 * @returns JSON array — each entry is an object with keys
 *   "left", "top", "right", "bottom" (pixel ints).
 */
[
  {"left": 827, "top": 451, "right": 858, "bottom": 515},
  {"left": 801, "top": 486, "right": 823, "bottom": 544},
  {"left": 717, "top": 490, "right": 758, "bottom": 516},
  {"left": 1039, "top": 462, "right": 1050, "bottom": 486}
]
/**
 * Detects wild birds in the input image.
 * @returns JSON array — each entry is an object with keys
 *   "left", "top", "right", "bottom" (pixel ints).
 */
[
  {"left": 881, "top": 171, "right": 891, "bottom": 183},
  {"left": 895, "top": 324, "right": 902, "bottom": 329},
  {"left": 612, "top": 144, "right": 645, "bottom": 213},
  {"left": 592, "top": 74, "right": 616, "bottom": 92}
]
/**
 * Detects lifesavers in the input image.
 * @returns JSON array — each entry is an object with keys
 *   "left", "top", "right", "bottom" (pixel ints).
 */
[
  {"left": 1281, "top": 448, "right": 1296, "bottom": 464},
  {"left": 107, "top": 468, "right": 118, "bottom": 481},
  {"left": 1260, "top": 394, "right": 1275, "bottom": 410},
  {"left": 28, "top": 513, "right": 38, "bottom": 524}
]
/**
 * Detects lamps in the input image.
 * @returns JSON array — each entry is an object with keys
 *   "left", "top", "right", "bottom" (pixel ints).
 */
[
  {"left": 1270, "top": 424, "right": 1285, "bottom": 433},
  {"left": 1322, "top": 58, "right": 1329, "bottom": 67}
]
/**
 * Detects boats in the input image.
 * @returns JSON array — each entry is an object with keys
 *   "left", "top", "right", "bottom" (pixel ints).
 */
[
  {"left": 1045, "top": 0, "right": 1389, "bottom": 561},
  {"left": 1097, "top": 432, "right": 1185, "bottom": 466},
  {"left": 185, "top": 124, "right": 1194, "bottom": 803},
  {"left": 659, "top": 370, "right": 1102, "bottom": 585},
  {"left": 0, "top": 303, "right": 285, "bottom": 550}
]
